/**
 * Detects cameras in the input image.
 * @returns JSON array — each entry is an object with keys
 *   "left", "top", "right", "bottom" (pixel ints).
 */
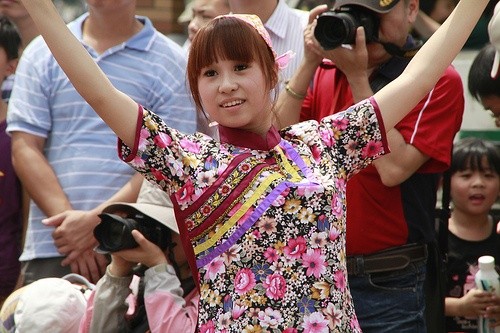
[
  {"left": 93, "top": 213, "right": 172, "bottom": 254},
  {"left": 314, "top": 5, "right": 378, "bottom": 50}
]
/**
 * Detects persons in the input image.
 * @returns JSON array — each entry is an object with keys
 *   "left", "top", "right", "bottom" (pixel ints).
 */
[{"left": 0, "top": 1, "right": 498, "bottom": 332}]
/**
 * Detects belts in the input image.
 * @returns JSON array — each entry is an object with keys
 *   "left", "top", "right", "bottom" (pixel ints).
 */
[{"left": 346, "top": 243, "right": 429, "bottom": 276}]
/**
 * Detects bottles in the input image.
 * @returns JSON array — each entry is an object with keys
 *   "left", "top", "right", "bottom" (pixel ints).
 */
[{"left": 474, "top": 256, "right": 500, "bottom": 333}]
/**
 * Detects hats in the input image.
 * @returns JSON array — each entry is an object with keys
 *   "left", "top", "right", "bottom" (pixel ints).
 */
[
  {"left": 0, "top": 273, "right": 96, "bottom": 333},
  {"left": 100, "top": 173, "right": 181, "bottom": 239},
  {"left": 488, "top": 0, "right": 500, "bottom": 79},
  {"left": 178, "top": 0, "right": 193, "bottom": 22},
  {"left": 329, "top": 0, "right": 400, "bottom": 14},
  {"left": 213, "top": 12, "right": 296, "bottom": 70}
]
